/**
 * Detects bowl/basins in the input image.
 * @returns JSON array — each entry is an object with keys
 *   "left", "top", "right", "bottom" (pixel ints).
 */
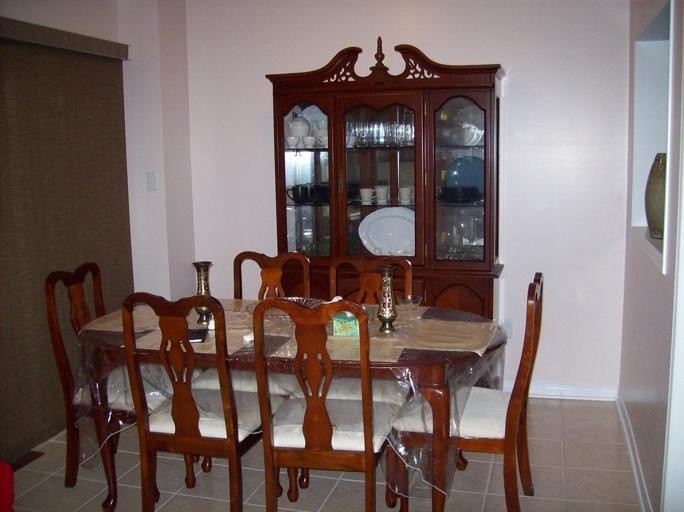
[
  {"left": 435, "top": 123, "right": 484, "bottom": 147},
  {"left": 397, "top": 295, "right": 423, "bottom": 310}
]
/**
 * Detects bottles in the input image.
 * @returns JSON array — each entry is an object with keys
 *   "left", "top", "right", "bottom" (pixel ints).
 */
[{"left": 286, "top": 112, "right": 311, "bottom": 149}]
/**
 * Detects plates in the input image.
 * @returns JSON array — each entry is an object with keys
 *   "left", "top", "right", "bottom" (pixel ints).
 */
[
  {"left": 244, "top": 296, "right": 326, "bottom": 328},
  {"left": 358, "top": 207, "right": 416, "bottom": 258},
  {"left": 437, "top": 153, "right": 484, "bottom": 204}
]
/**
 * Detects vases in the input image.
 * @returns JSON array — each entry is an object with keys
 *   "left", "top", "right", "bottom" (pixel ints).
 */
[{"left": 645, "top": 153, "right": 666, "bottom": 239}]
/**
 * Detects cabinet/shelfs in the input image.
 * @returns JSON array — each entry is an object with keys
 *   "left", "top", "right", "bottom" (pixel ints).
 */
[{"left": 265, "top": 36, "right": 505, "bottom": 388}]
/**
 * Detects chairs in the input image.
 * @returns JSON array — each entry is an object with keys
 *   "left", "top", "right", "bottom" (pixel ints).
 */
[
  {"left": 46, "top": 262, "right": 191, "bottom": 509},
  {"left": 253, "top": 299, "right": 401, "bottom": 510},
  {"left": 122, "top": 292, "right": 286, "bottom": 512},
  {"left": 291, "top": 256, "right": 413, "bottom": 489},
  {"left": 204, "top": 251, "right": 312, "bottom": 472},
  {"left": 393, "top": 271, "right": 543, "bottom": 511}
]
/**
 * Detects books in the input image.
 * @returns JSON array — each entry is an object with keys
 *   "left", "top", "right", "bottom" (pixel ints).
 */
[{"left": 179, "top": 328, "right": 208, "bottom": 342}]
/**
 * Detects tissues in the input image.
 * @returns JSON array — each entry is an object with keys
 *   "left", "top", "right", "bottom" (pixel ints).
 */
[{"left": 323, "top": 296, "right": 371, "bottom": 337}]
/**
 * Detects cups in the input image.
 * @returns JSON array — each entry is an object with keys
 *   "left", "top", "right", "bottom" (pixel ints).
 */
[
  {"left": 285, "top": 182, "right": 329, "bottom": 206},
  {"left": 345, "top": 119, "right": 414, "bottom": 148},
  {"left": 284, "top": 134, "right": 329, "bottom": 149},
  {"left": 359, "top": 184, "right": 414, "bottom": 205}
]
